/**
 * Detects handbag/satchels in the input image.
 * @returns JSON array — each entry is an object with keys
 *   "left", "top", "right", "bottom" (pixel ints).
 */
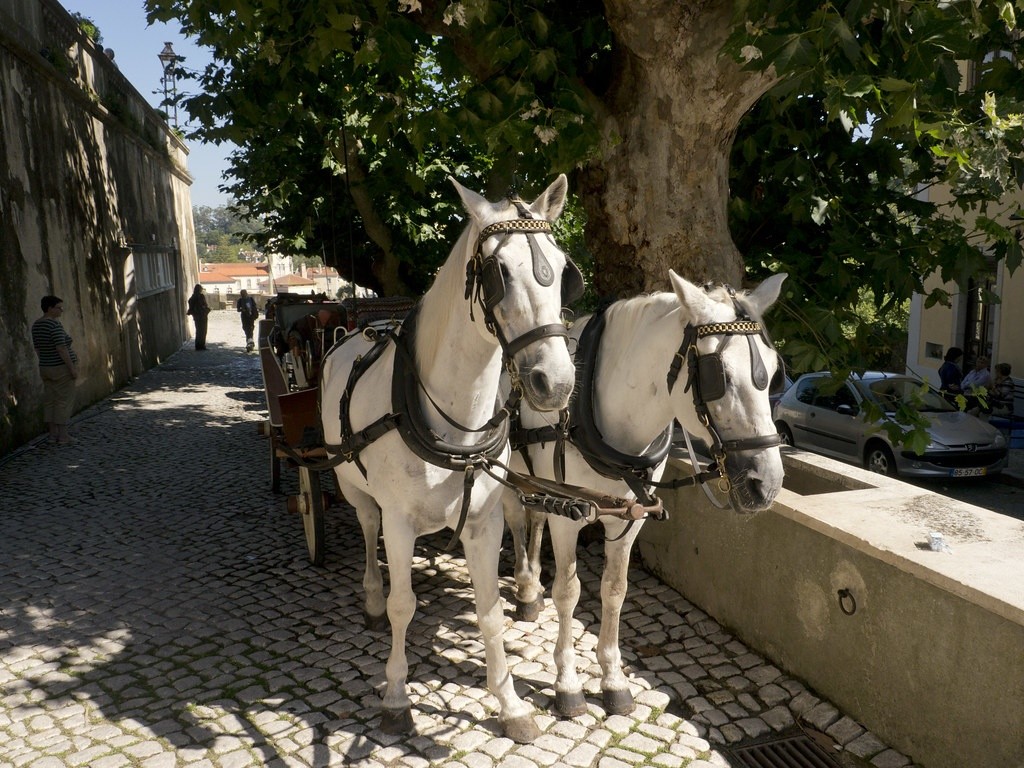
[{"left": 250, "top": 297, "right": 258, "bottom": 319}]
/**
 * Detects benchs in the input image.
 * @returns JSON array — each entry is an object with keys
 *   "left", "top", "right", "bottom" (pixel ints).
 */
[
  {"left": 274, "top": 297, "right": 417, "bottom": 340},
  {"left": 987, "top": 385, "right": 1024, "bottom": 444}
]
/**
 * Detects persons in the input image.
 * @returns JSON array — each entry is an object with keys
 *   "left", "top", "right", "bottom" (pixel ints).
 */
[
  {"left": 265, "top": 298, "right": 274, "bottom": 319},
  {"left": 186, "top": 284, "right": 210, "bottom": 351},
  {"left": 237, "top": 290, "right": 258, "bottom": 352},
  {"left": 937, "top": 347, "right": 1015, "bottom": 446},
  {"left": 32, "top": 295, "right": 77, "bottom": 446}
]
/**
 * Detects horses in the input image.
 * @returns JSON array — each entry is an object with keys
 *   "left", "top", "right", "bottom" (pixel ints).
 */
[{"left": 317, "top": 171, "right": 792, "bottom": 745}]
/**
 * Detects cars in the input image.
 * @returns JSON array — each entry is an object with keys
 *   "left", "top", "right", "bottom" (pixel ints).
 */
[
  {"left": 772, "top": 370, "right": 1010, "bottom": 485},
  {"left": 769, "top": 373, "right": 809, "bottom": 415}
]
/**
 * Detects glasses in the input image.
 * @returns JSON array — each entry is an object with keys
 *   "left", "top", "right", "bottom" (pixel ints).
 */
[{"left": 55, "top": 306, "right": 63, "bottom": 310}]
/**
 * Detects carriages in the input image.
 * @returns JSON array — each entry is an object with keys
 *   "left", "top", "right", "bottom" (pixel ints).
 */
[{"left": 255, "top": 168, "right": 793, "bottom": 748}]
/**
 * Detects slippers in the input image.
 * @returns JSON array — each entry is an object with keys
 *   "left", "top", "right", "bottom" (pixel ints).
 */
[
  {"left": 48, "top": 439, "right": 58, "bottom": 443},
  {"left": 59, "top": 437, "right": 81, "bottom": 444}
]
